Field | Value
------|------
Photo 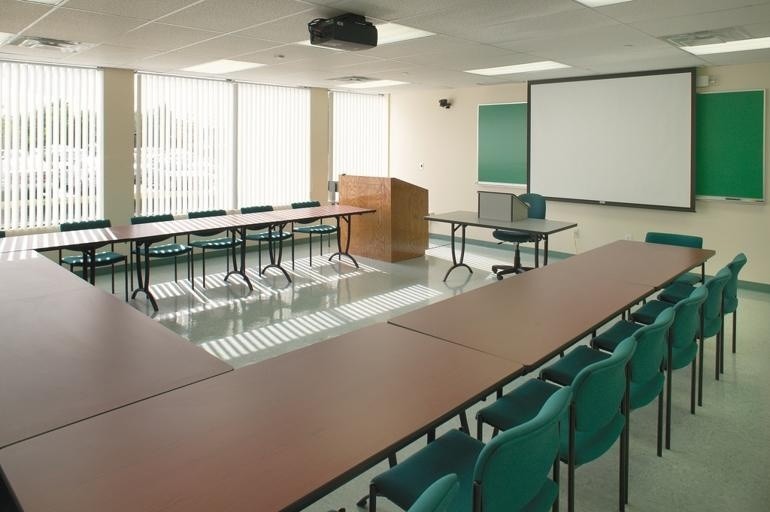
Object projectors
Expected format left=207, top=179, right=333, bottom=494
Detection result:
left=307, top=13, right=378, bottom=52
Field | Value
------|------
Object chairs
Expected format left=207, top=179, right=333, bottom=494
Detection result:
left=292, top=202, right=340, bottom=267
left=59, top=220, right=128, bottom=301
left=188, top=210, right=243, bottom=288
left=130, top=214, right=194, bottom=297
left=241, top=206, right=295, bottom=276
left=492, top=194, right=546, bottom=280
left=646, top=232, right=703, bottom=287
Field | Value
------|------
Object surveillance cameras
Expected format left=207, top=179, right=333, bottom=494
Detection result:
left=439, top=99, right=447, bottom=107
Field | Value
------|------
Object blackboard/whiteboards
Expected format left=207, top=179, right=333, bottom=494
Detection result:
left=477, top=88, right=766, bottom=204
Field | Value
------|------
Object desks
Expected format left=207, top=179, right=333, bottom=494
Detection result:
left=0, top=250, right=233, bottom=449
left=0, top=203, right=377, bottom=311
left=387, top=240, right=717, bottom=401
left=1, top=323, right=525, bottom=512
left=424, top=210, right=577, bottom=282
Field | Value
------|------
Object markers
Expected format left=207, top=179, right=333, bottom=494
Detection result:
left=756, top=201, right=763, bottom=202
left=726, top=198, right=740, bottom=200
